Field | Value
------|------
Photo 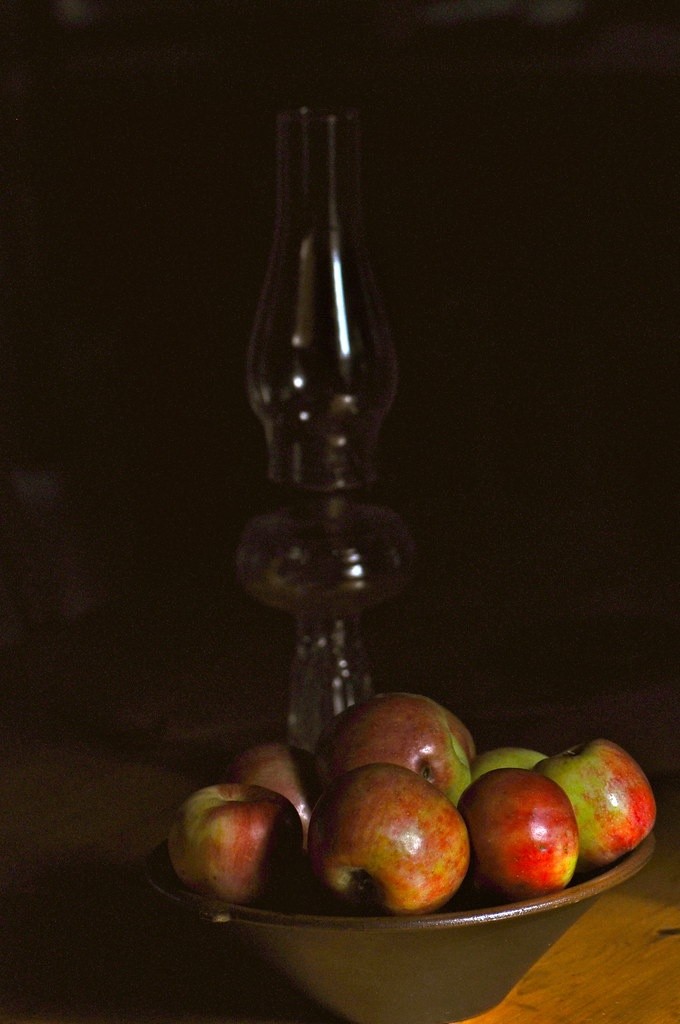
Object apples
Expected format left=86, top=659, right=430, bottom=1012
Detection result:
left=165, top=740, right=317, bottom=904
left=307, top=691, right=656, bottom=919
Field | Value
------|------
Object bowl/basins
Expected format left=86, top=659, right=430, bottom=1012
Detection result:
left=145, top=830, right=657, bottom=1024
